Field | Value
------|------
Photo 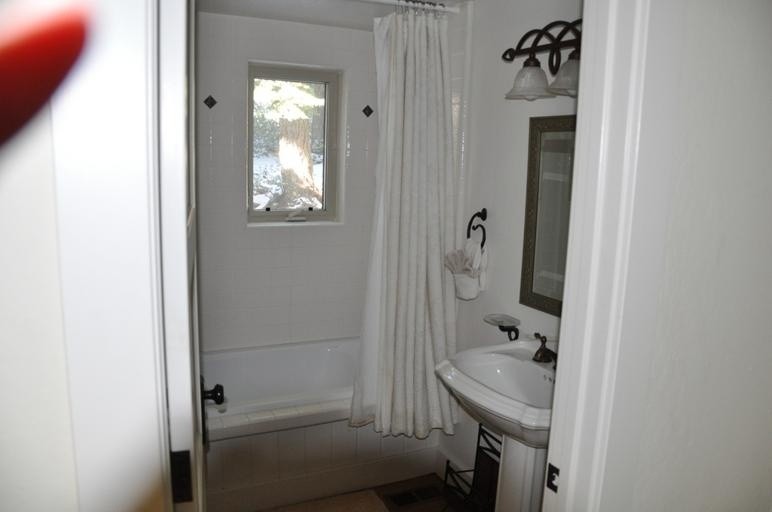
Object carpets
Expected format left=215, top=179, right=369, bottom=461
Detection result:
left=271, top=490, right=389, bottom=512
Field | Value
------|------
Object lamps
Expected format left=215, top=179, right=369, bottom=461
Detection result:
left=501, top=19, right=582, bottom=101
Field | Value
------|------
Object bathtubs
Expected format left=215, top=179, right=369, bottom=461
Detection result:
left=202, top=336, right=442, bottom=508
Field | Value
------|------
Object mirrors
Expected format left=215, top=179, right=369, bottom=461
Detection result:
left=519, top=114, right=577, bottom=318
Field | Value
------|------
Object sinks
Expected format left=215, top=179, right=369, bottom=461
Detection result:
left=434, top=339, right=557, bottom=447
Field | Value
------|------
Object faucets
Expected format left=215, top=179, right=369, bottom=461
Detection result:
left=534, top=332, right=551, bottom=362
